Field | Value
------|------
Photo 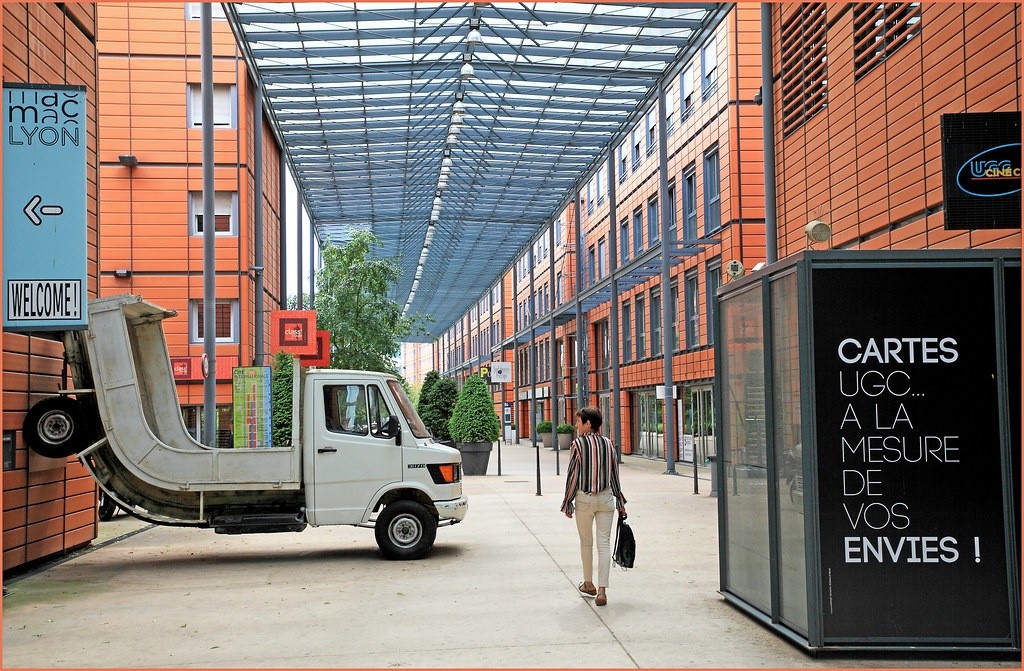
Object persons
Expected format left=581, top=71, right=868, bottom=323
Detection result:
left=559, top=406, right=627, bottom=607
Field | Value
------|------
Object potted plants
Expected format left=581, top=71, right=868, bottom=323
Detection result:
left=537, top=421, right=553, bottom=448
left=417, top=370, right=500, bottom=476
left=556, top=424, right=574, bottom=450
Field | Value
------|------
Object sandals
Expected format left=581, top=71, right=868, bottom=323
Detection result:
left=595, top=593, right=607, bottom=606
left=579, top=581, right=596, bottom=594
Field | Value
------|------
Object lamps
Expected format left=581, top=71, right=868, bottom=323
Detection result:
left=119, top=156, right=138, bottom=166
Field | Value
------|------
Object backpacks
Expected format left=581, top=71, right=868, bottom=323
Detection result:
left=612, top=511, right=635, bottom=571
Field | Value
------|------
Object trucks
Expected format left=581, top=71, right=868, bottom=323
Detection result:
left=22, top=290, right=472, bottom=560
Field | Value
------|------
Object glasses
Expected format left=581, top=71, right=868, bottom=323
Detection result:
left=581, top=408, right=588, bottom=416
